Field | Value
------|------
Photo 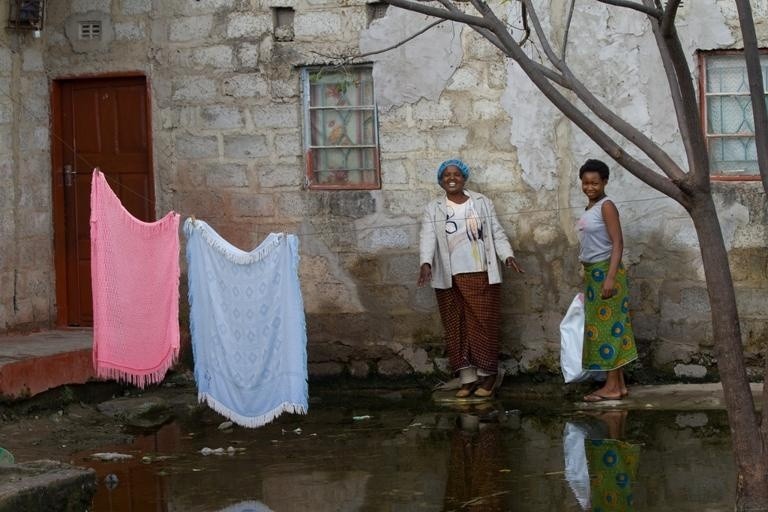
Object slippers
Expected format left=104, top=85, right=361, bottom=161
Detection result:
left=583, top=391, right=629, bottom=402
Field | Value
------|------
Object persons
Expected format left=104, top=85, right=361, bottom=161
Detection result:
left=578, top=408, right=642, bottom=511
left=416, top=159, right=526, bottom=399
left=574, top=157, right=640, bottom=402
left=438, top=426, right=507, bottom=511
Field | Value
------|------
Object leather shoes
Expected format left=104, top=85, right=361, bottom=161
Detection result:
left=474, top=378, right=497, bottom=396
left=455, top=384, right=476, bottom=397
